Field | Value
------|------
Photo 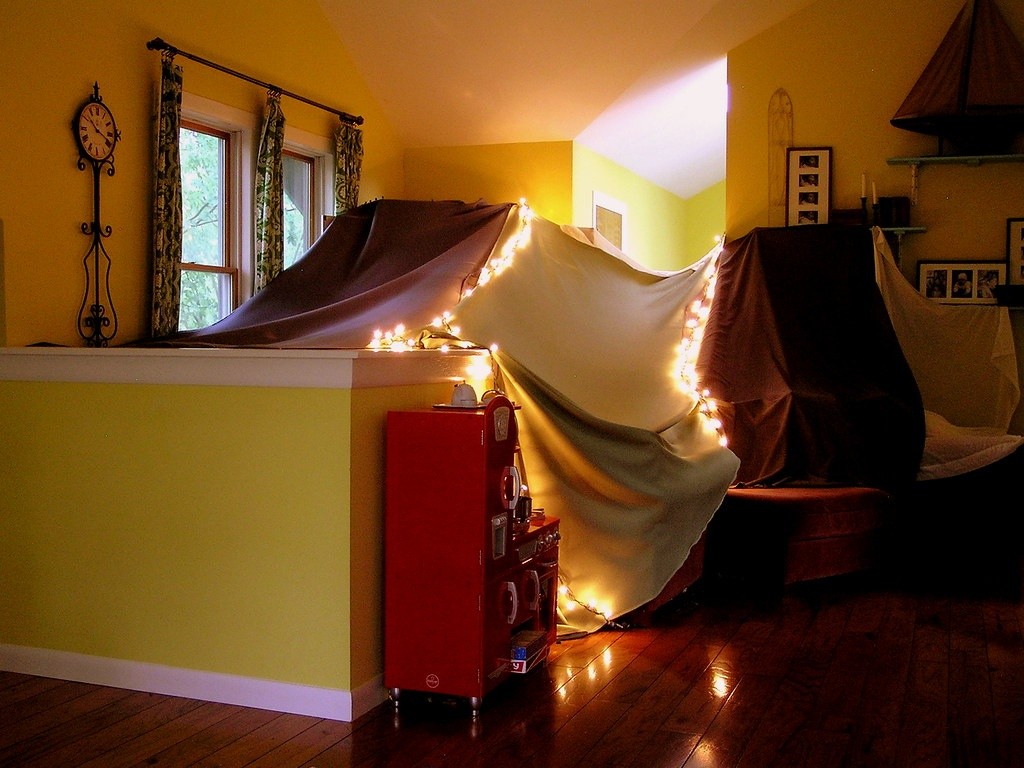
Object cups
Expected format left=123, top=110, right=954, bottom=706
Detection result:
left=516, top=496, right=532, bottom=519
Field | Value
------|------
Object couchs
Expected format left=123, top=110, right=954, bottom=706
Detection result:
left=640, top=484, right=885, bottom=611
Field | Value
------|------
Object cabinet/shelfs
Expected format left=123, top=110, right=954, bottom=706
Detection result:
left=385, top=392, right=562, bottom=728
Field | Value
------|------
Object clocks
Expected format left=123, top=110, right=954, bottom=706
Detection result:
left=71, top=81, right=122, bottom=347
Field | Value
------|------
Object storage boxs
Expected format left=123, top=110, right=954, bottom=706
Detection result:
left=511, top=631, right=548, bottom=674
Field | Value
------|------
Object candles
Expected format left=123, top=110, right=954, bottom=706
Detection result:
left=862, top=172, right=866, bottom=196
left=872, top=180, right=877, bottom=204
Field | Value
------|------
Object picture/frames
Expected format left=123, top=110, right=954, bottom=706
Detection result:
left=784, top=146, right=832, bottom=227
left=916, top=260, right=1009, bottom=306
left=1006, top=217, right=1024, bottom=287
